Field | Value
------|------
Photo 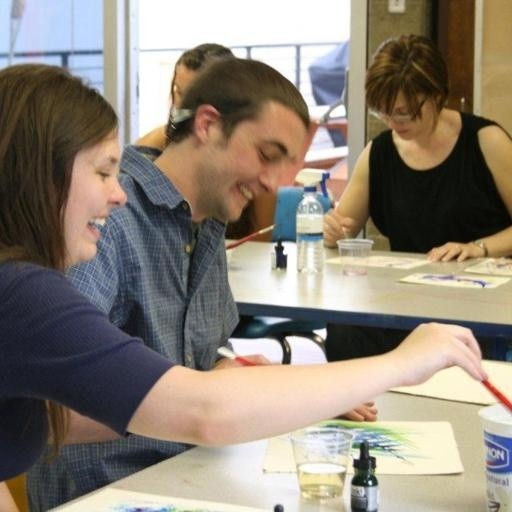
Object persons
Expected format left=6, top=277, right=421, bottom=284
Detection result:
left=134, top=43, right=277, bottom=243
left=0, top=64, right=488, bottom=512
left=323, top=34, right=512, bottom=360
left=26, top=58, right=378, bottom=508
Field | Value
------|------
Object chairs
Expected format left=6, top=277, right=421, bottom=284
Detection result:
left=272, top=188, right=332, bottom=242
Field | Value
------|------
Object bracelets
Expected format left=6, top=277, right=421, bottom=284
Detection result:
left=472, top=240, right=488, bottom=257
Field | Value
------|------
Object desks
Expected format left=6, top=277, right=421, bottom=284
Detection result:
left=47, top=359, right=512, bottom=511
left=222, top=239, right=512, bottom=341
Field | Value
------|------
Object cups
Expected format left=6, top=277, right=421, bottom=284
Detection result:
left=293, top=429, right=354, bottom=500
left=336, top=237, right=371, bottom=277
left=478, top=402, right=511, bottom=512
left=226, top=239, right=238, bottom=263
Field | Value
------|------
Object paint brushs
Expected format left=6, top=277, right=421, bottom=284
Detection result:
left=331, top=202, right=355, bottom=256
left=481, top=379, right=512, bottom=411
left=225, top=223, right=278, bottom=250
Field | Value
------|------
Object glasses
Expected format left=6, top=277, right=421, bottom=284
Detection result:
left=369, top=97, right=429, bottom=122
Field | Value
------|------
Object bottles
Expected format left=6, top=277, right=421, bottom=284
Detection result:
left=294, top=183, right=324, bottom=275
left=352, top=440, right=379, bottom=511
left=270, top=235, right=287, bottom=269
